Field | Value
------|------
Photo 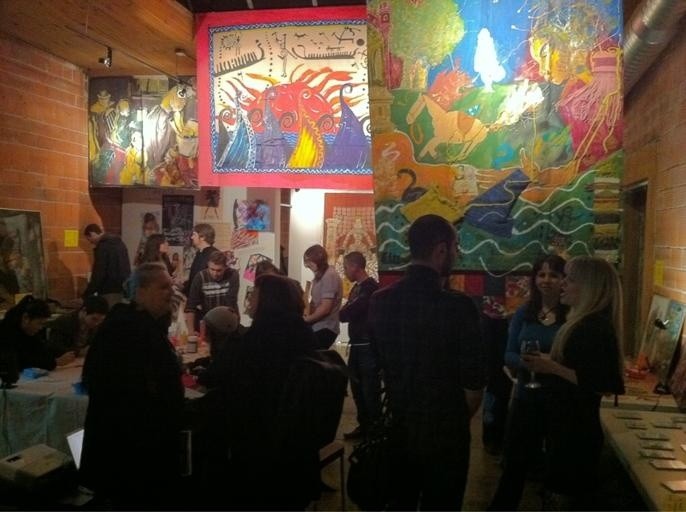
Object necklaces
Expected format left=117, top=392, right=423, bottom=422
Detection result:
left=535, top=304, right=559, bottom=320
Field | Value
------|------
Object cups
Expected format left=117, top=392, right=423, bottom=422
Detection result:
left=520, top=341, right=542, bottom=389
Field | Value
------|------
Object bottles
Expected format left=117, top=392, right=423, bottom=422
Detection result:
left=187, top=336, right=198, bottom=353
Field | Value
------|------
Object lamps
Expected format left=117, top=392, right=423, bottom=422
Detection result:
left=176, top=81, right=186, bottom=99
left=99, top=55, right=111, bottom=69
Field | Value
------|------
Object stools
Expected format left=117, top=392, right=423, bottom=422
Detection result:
left=1, top=444, right=72, bottom=510
left=315, top=439, right=345, bottom=510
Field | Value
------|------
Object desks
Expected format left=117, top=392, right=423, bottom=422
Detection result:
left=4, top=338, right=216, bottom=478
left=599, top=352, right=680, bottom=411
left=597, top=407, right=686, bottom=512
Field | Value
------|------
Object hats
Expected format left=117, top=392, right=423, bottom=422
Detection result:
left=202, top=305, right=238, bottom=335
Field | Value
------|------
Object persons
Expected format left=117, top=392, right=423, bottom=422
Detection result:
left=0, top=295, right=73, bottom=383
left=140, top=231, right=179, bottom=278
left=522, top=258, right=626, bottom=507
left=300, top=242, right=343, bottom=346
left=247, top=260, right=282, bottom=277
left=46, top=294, right=108, bottom=357
left=338, top=247, right=380, bottom=440
left=74, top=224, right=130, bottom=309
left=488, top=254, right=570, bottom=510
left=183, top=250, right=240, bottom=338
left=191, top=302, right=246, bottom=384
left=366, top=211, right=495, bottom=509
left=184, top=223, right=220, bottom=298
left=77, top=260, right=184, bottom=512
left=213, top=272, right=347, bottom=512
